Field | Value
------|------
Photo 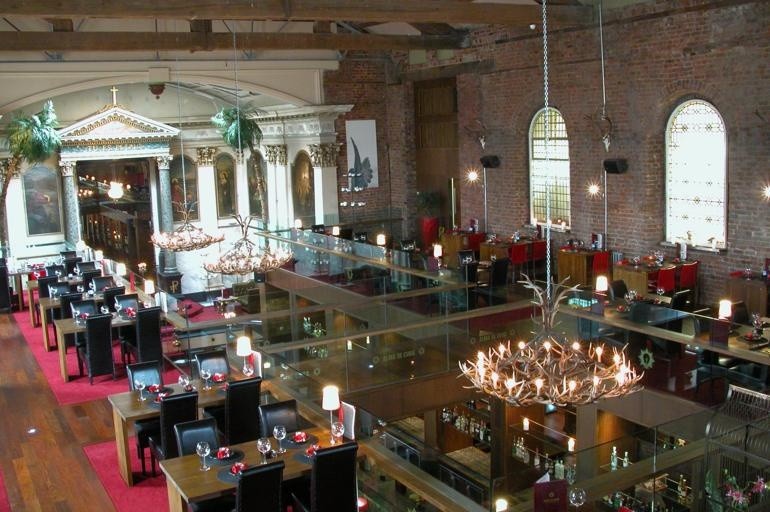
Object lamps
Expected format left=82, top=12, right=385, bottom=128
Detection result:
left=201, top=18, right=294, bottom=275
left=462, top=0, right=645, bottom=412
left=142, top=19, right=230, bottom=250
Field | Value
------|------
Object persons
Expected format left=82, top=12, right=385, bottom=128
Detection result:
left=171, top=178, right=183, bottom=216
left=218, top=171, right=233, bottom=214
left=185, top=191, right=197, bottom=214
left=250, top=171, right=268, bottom=210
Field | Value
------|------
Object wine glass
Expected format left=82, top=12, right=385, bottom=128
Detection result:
left=332, top=421, right=345, bottom=444
left=202, top=369, right=212, bottom=391
left=31, top=255, right=123, bottom=322
left=244, top=364, right=253, bottom=378
left=273, top=425, right=287, bottom=456
left=196, top=442, right=210, bottom=473
left=257, top=436, right=274, bottom=466
left=177, top=376, right=192, bottom=392
left=134, top=379, right=147, bottom=402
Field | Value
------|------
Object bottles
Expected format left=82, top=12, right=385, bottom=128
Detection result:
left=607, top=492, right=672, bottom=511
left=675, top=473, right=688, bottom=501
left=608, top=445, right=618, bottom=466
left=442, top=399, right=491, bottom=442
left=621, top=449, right=629, bottom=467
left=512, top=435, right=577, bottom=485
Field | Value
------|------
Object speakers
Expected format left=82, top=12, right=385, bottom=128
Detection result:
left=480, top=155, right=500, bottom=168
left=604, top=158, right=628, bottom=174
